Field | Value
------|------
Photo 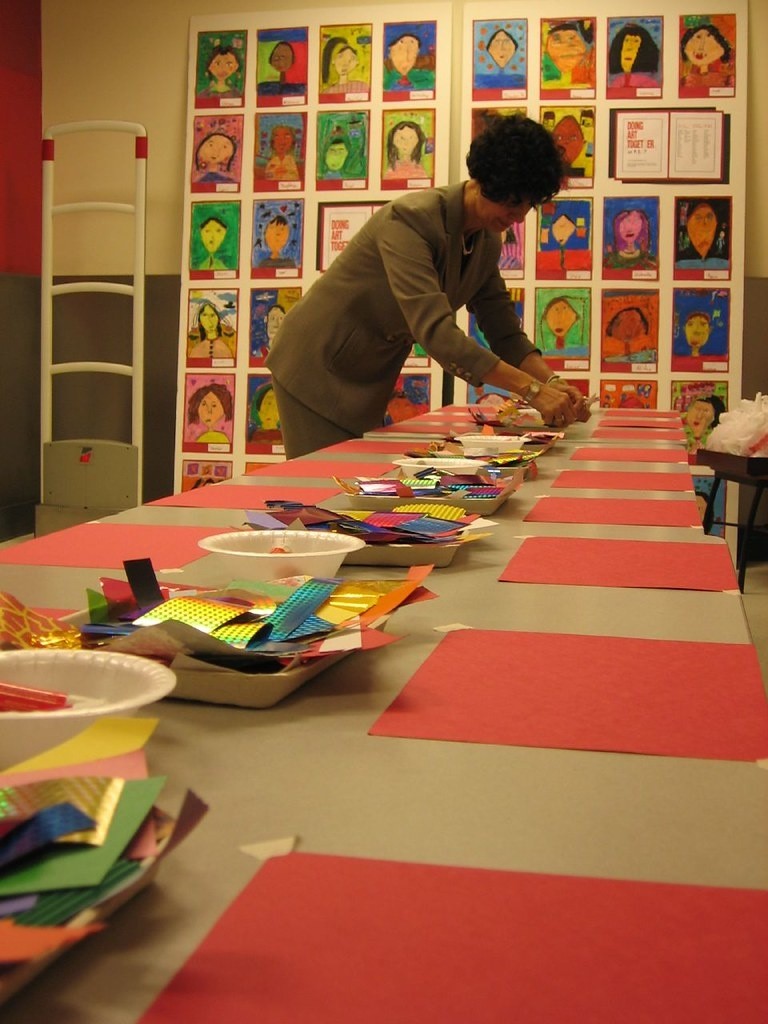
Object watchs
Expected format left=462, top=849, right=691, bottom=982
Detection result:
left=523, top=381, right=541, bottom=405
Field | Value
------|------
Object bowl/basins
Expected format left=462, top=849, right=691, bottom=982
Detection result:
left=198, top=528, right=366, bottom=580
left=392, top=458, right=488, bottom=479
left=0, top=648, right=178, bottom=774
left=454, top=435, right=533, bottom=454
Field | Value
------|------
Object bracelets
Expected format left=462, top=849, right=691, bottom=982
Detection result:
left=547, top=375, right=560, bottom=384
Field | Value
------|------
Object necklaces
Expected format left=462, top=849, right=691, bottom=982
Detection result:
left=461, top=234, right=475, bottom=254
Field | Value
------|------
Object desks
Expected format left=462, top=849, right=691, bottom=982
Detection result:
left=0, top=404, right=768, bottom=1024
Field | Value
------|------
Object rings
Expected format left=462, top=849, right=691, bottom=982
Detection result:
left=583, top=403, right=586, bottom=409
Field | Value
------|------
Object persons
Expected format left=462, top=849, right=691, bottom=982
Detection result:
left=262, top=112, right=592, bottom=466
